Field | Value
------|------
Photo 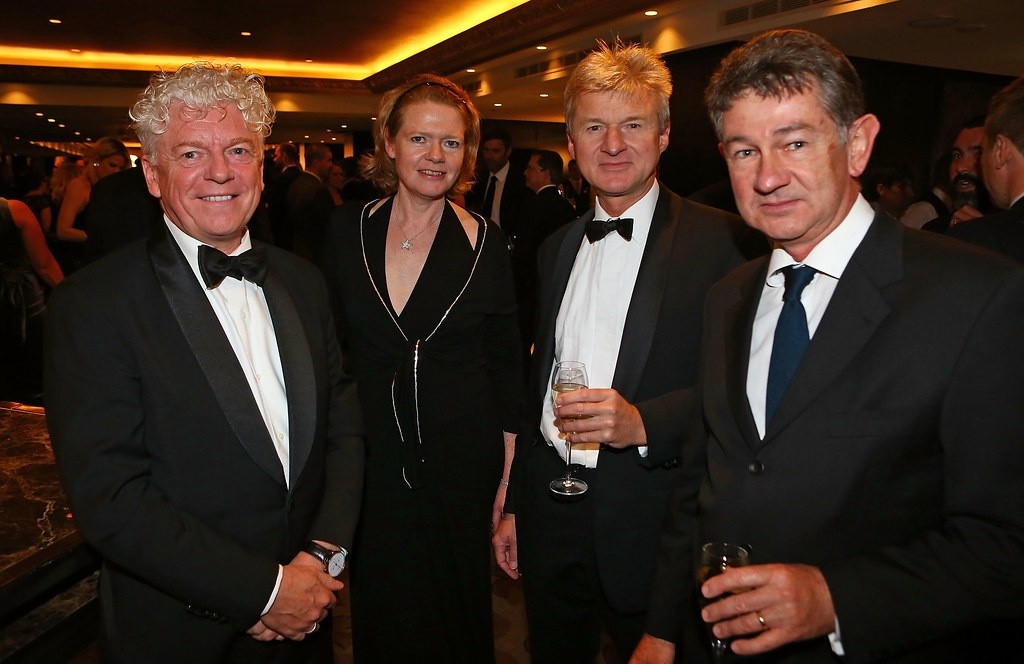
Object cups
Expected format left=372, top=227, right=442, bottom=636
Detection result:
left=509, top=233, right=520, bottom=256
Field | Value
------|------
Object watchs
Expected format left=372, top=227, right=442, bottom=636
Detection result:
left=301, top=540, right=346, bottom=579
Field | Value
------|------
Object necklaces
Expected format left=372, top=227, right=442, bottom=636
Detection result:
left=394, top=195, right=445, bottom=250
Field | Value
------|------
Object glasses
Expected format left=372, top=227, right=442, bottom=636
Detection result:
left=526, top=165, right=543, bottom=171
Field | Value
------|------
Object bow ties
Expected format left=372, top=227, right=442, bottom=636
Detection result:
left=585, top=218, right=633, bottom=244
left=198, top=244, right=269, bottom=290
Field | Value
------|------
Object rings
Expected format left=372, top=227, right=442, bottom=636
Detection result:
left=757, top=610, right=769, bottom=631
left=304, top=623, right=317, bottom=635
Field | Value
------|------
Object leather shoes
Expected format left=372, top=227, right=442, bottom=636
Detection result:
left=33, top=391, right=41, bottom=400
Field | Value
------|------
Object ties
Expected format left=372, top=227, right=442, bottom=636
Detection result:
left=482, top=176, right=498, bottom=218
left=766, top=266, right=818, bottom=434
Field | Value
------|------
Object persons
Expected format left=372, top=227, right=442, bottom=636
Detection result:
left=0, top=135, right=591, bottom=406
left=902, top=114, right=987, bottom=232
left=947, top=72, right=1024, bottom=264
left=868, top=166, right=914, bottom=226
left=494, top=34, right=761, bottom=664
left=42, top=62, right=365, bottom=664
left=627, top=26, right=1024, bottom=664
left=314, top=72, right=520, bottom=663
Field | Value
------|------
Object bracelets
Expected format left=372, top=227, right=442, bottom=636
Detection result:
left=500, top=479, right=508, bottom=485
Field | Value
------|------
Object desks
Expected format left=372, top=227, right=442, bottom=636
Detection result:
left=0, top=398, right=100, bottom=663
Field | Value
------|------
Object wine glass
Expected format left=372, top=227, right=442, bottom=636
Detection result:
left=699, top=542, right=752, bottom=664
left=550, top=361, right=588, bottom=495
